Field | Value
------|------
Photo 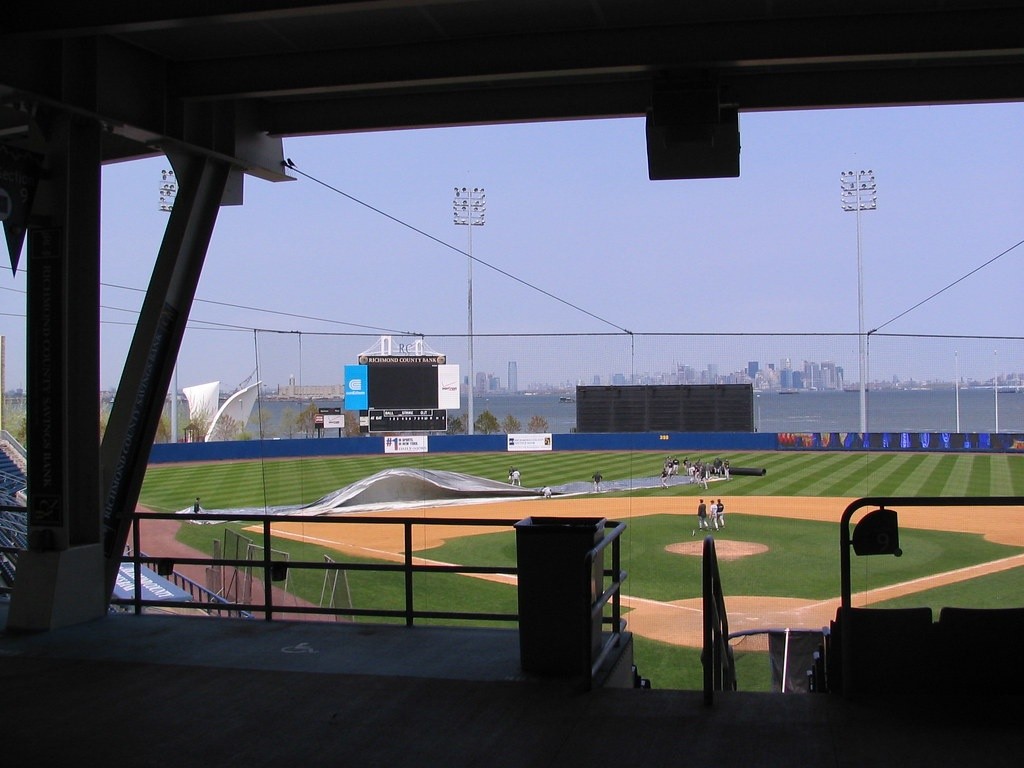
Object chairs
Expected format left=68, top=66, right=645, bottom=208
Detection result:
left=810, top=607, right=1024, bottom=695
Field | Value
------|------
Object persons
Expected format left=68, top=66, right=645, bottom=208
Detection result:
left=194, top=496, right=200, bottom=514
left=592, top=471, right=603, bottom=492
left=660, top=456, right=730, bottom=489
left=698, top=499, right=726, bottom=532
left=508, top=465, right=521, bottom=486
left=541, top=486, right=551, bottom=498
